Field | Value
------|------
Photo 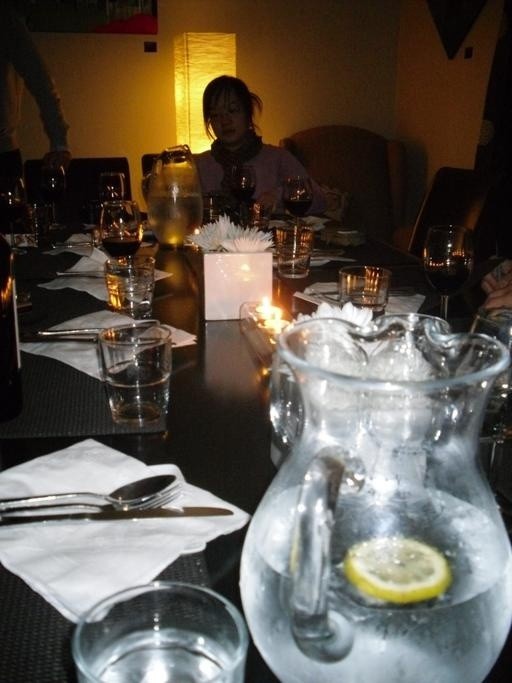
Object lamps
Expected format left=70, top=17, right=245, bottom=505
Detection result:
left=173, top=29, right=238, bottom=153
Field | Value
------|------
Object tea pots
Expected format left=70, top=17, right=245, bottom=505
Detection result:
left=239, top=311, right=512, bottom=683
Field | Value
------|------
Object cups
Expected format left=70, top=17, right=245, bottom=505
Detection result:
left=457, top=309, right=511, bottom=415
left=71, top=580, right=248, bottom=680
left=93, top=323, right=173, bottom=429
left=104, top=255, right=156, bottom=318
left=338, top=265, right=391, bottom=319
left=276, top=224, right=313, bottom=278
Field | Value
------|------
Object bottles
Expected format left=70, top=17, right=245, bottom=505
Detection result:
left=146, top=145, right=204, bottom=249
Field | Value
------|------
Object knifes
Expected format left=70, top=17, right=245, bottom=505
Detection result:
left=1, top=506, right=234, bottom=525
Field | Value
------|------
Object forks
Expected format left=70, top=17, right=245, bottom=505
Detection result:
left=0, top=482, right=183, bottom=511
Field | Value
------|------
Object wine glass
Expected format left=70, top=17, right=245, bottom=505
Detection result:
left=99, top=200, right=143, bottom=265
left=33, top=162, right=68, bottom=232
left=1, top=174, right=27, bottom=256
left=280, top=173, right=314, bottom=225
left=422, top=224, right=473, bottom=323
left=95, top=172, right=125, bottom=200
left=228, top=163, right=256, bottom=220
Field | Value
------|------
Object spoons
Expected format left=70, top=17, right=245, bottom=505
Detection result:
left=1, top=473, right=176, bottom=507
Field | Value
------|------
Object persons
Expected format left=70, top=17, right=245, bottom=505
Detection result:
left=195, top=75, right=328, bottom=216
left=0, top=0, right=72, bottom=291
left=480, top=259, right=512, bottom=310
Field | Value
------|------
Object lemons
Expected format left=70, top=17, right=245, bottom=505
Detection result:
left=343, top=535, right=452, bottom=606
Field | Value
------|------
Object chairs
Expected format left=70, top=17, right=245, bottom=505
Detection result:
left=276, top=124, right=407, bottom=270
left=141, top=152, right=223, bottom=233
left=22, top=156, right=131, bottom=232
left=408, top=164, right=502, bottom=272
